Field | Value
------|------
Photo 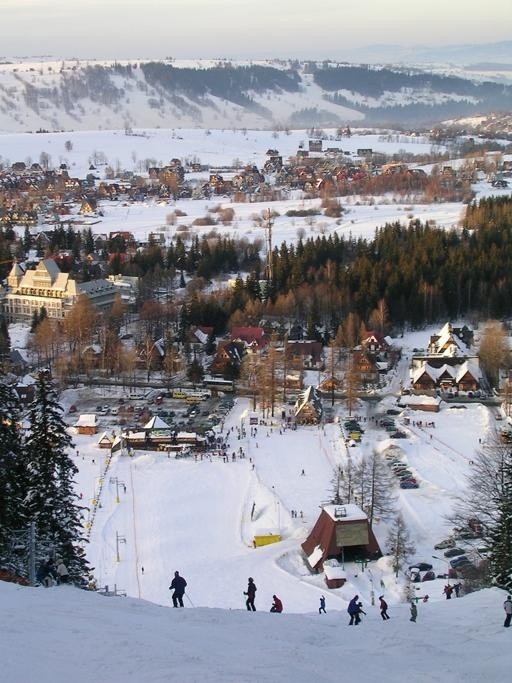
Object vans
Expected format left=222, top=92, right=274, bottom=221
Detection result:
left=127, top=394, right=142, bottom=399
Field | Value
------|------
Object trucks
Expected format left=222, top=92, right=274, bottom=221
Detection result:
left=453, top=519, right=484, bottom=538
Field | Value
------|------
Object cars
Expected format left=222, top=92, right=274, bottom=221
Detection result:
left=420, top=571, right=435, bottom=581
left=158, top=410, right=176, bottom=423
left=96, top=404, right=118, bottom=415
left=409, top=562, right=432, bottom=571
left=344, top=420, right=364, bottom=439
left=182, top=406, right=209, bottom=424
left=434, top=539, right=472, bottom=571
left=379, top=418, right=406, bottom=439
left=391, top=463, right=418, bottom=488
left=70, top=406, right=75, bottom=412
left=386, top=409, right=399, bottom=415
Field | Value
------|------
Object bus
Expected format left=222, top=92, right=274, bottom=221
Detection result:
left=172, top=392, right=207, bottom=404
left=204, top=380, right=233, bottom=392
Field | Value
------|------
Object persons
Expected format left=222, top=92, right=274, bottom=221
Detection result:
left=269, top=594, right=283, bottom=613
left=243, top=576, right=258, bottom=611
left=454, top=583, right=459, bottom=597
left=194, top=423, right=286, bottom=469
left=444, top=585, right=451, bottom=599
left=378, top=593, right=390, bottom=621
left=357, top=601, right=366, bottom=621
left=503, top=594, right=511, bottom=627
left=347, top=594, right=360, bottom=625
left=409, top=601, right=416, bottom=622
left=318, top=594, right=326, bottom=613
left=169, top=570, right=187, bottom=607
